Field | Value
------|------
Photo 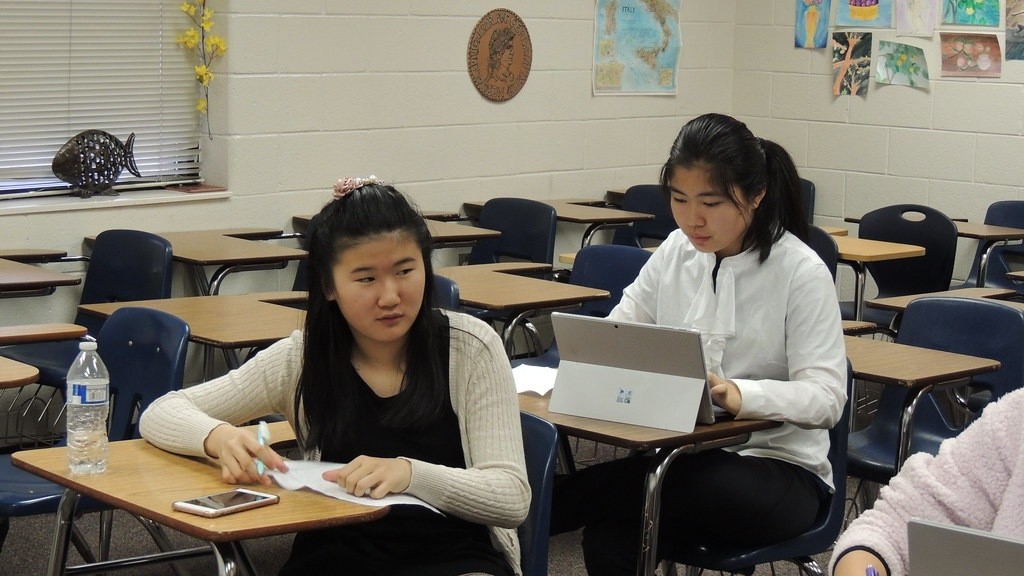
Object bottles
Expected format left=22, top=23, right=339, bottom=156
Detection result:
left=67, top=342, right=110, bottom=474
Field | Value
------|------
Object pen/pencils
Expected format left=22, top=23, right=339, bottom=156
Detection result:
left=254, top=421, right=271, bottom=476
left=866, top=565, right=876, bottom=576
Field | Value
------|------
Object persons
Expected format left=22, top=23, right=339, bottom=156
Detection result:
left=136, top=177, right=533, bottom=576
left=827, top=382, right=1024, bottom=576
left=550, top=113, right=851, bottom=576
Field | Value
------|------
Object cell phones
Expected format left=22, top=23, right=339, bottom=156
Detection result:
left=171, top=488, right=279, bottom=517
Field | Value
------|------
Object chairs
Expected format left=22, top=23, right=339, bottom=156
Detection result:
left=848, top=296, right=1024, bottom=496
left=0, top=229, right=174, bottom=449
left=0, top=307, right=259, bottom=576
left=838, top=204, right=958, bottom=336
left=660, top=356, right=852, bottom=576
left=580, top=183, right=681, bottom=250
left=511, top=245, right=652, bottom=371
left=782, top=176, right=837, bottom=284
left=468, top=197, right=557, bottom=357
left=949, top=199, right=1024, bottom=301
left=520, top=410, right=558, bottom=576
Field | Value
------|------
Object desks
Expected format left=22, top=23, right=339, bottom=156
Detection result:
left=0, top=201, right=1024, bottom=576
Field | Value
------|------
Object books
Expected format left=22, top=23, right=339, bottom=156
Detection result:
left=163, top=184, right=228, bottom=193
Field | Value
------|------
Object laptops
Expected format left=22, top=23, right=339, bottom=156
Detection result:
left=551, top=312, right=735, bottom=425
left=907, top=518, right=1024, bottom=576
